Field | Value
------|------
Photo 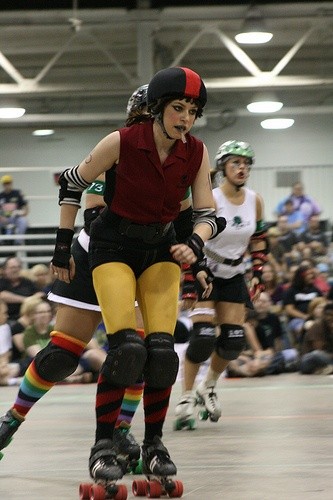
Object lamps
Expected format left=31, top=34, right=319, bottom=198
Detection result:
left=246, top=91, right=284, bottom=113
left=235, top=5, right=274, bottom=43
left=0, top=98, right=26, bottom=118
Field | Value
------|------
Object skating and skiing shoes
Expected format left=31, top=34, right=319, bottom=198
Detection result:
left=78, top=445, right=128, bottom=500
left=112, top=428, right=144, bottom=475
left=132, top=440, right=184, bottom=498
left=0, top=410, right=21, bottom=462
left=196, top=382, right=222, bottom=422
left=173, top=394, right=195, bottom=429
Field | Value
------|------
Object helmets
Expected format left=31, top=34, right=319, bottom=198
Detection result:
left=127, top=84, right=149, bottom=116
left=215, top=140, right=255, bottom=166
left=147, top=67, right=208, bottom=107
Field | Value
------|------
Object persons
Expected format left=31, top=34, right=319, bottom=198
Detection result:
left=0, top=83, right=215, bottom=475
left=0, top=175, right=28, bottom=245
left=50, top=67, right=218, bottom=500
left=174, top=140, right=265, bottom=431
left=173, top=182, right=333, bottom=377
left=0, top=256, right=110, bottom=385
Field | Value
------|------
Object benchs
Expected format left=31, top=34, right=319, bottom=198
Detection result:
left=0, top=234, right=79, bottom=270
